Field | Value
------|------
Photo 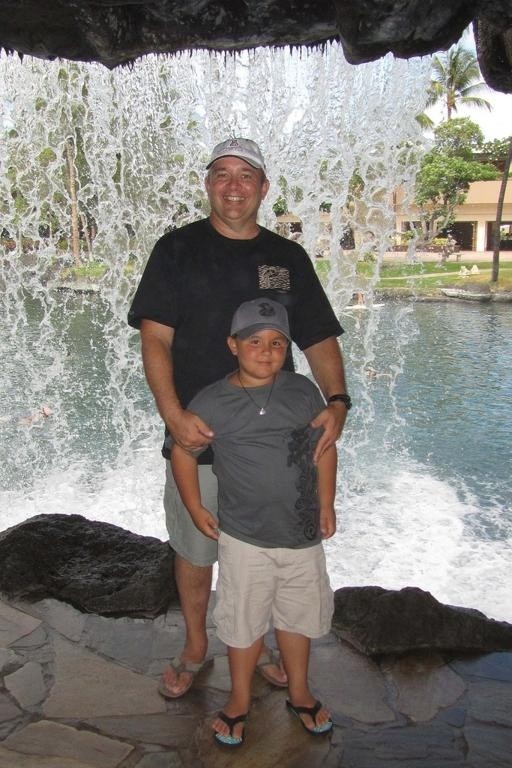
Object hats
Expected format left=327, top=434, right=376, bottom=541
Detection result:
left=230, top=297, right=291, bottom=342
left=205, top=138, right=264, bottom=170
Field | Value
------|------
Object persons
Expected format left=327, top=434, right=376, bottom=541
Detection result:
left=125, top=135, right=354, bottom=701
left=168, top=295, right=338, bottom=746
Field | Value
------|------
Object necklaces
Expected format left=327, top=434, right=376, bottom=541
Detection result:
left=234, top=369, right=277, bottom=416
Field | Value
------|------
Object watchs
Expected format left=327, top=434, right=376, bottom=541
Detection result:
left=326, top=392, right=353, bottom=410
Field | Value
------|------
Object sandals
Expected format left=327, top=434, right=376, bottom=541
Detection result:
left=285, top=696, right=333, bottom=734
left=159, top=655, right=215, bottom=698
left=213, top=711, right=248, bottom=747
left=255, top=651, right=288, bottom=687
